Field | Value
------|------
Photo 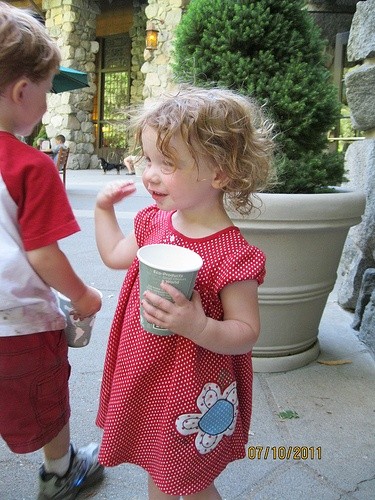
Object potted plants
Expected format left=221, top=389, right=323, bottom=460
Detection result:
left=166, top=0, right=369, bottom=374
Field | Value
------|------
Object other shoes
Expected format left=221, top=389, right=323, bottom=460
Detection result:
left=126, top=172, right=135, bottom=175
left=38, top=441, right=105, bottom=500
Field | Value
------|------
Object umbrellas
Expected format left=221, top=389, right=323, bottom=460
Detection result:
left=47, top=65, right=90, bottom=94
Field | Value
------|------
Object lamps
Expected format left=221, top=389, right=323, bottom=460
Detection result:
left=145, top=15, right=167, bottom=52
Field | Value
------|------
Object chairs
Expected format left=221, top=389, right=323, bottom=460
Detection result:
left=54, top=146, right=70, bottom=191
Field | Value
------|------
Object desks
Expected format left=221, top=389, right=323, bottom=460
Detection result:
left=39, top=148, right=54, bottom=154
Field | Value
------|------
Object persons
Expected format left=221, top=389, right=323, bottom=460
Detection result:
left=95, top=85, right=286, bottom=500
left=0, top=1, right=105, bottom=500
left=124, top=155, right=136, bottom=175
left=42, top=134, right=67, bottom=170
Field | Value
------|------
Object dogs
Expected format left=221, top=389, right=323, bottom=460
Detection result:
left=96, top=156, right=127, bottom=176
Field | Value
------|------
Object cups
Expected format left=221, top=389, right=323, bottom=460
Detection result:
left=137, top=244, right=204, bottom=336
left=58, top=285, right=102, bottom=348
